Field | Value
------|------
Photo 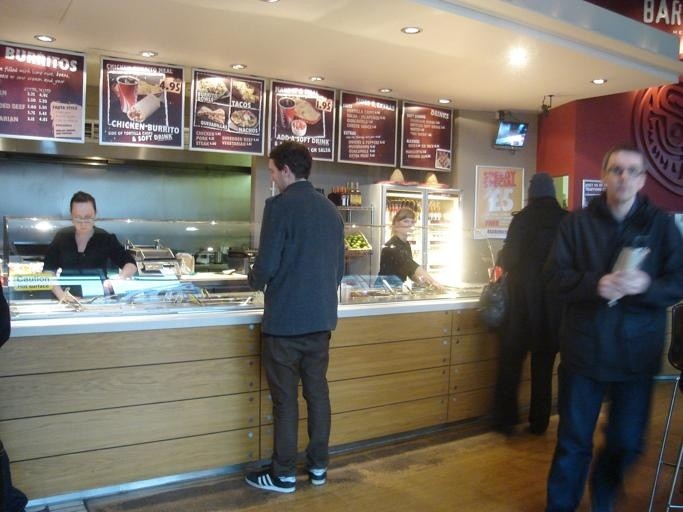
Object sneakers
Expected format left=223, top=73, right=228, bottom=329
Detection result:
left=244, top=472, right=297, bottom=493
left=481, top=283, right=506, bottom=326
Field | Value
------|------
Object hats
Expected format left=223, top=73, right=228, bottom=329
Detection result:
left=529, top=173, right=555, bottom=197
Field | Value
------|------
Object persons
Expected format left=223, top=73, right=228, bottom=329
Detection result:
left=0, top=281, right=29, bottom=512
left=541, top=144, right=682, bottom=511
left=42, top=191, right=139, bottom=304
left=374, top=208, right=438, bottom=289
left=476, top=172, right=574, bottom=435
left=242, top=140, right=345, bottom=494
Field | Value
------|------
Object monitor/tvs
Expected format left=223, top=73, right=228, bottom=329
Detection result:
left=492, top=120, right=528, bottom=149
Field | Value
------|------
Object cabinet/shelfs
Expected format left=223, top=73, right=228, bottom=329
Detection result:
left=335, top=204, right=374, bottom=289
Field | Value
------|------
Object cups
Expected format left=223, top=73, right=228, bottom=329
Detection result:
left=117, top=75, right=140, bottom=113
left=278, top=96, right=298, bottom=128
left=212, top=250, right=224, bottom=265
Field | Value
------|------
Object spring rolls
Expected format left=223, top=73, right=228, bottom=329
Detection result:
left=127, top=93, right=161, bottom=123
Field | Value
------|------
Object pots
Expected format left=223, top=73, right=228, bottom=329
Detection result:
left=227, top=243, right=259, bottom=275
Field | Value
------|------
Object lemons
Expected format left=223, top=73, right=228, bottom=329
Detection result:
left=345, top=232, right=369, bottom=250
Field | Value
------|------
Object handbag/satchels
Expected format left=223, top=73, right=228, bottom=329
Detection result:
left=309, top=468, right=326, bottom=486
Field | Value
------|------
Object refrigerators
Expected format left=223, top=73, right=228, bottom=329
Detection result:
left=348, top=182, right=465, bottom=290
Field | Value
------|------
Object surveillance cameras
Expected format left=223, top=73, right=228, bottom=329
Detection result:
left=542, top=104, right=551, bottom=114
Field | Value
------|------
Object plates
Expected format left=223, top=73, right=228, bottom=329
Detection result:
left=197, top=77, right=228, bottom=100
left=229, top=110, right=259, bottom=131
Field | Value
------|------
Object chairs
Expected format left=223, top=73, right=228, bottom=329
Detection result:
left=649, top=303, right=683, bottom=510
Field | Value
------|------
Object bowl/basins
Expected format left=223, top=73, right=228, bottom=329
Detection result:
left=290, top=118, right=306, bottom=137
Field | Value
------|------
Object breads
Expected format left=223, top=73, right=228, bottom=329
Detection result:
left=288, top=96, right=322, bottom=125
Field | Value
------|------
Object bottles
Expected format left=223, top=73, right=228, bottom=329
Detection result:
left=329, top=179, right=362, bottom=207
left=403, top=226, right=443, bottom=254
left=386, top=197, right=441, bottom=223
